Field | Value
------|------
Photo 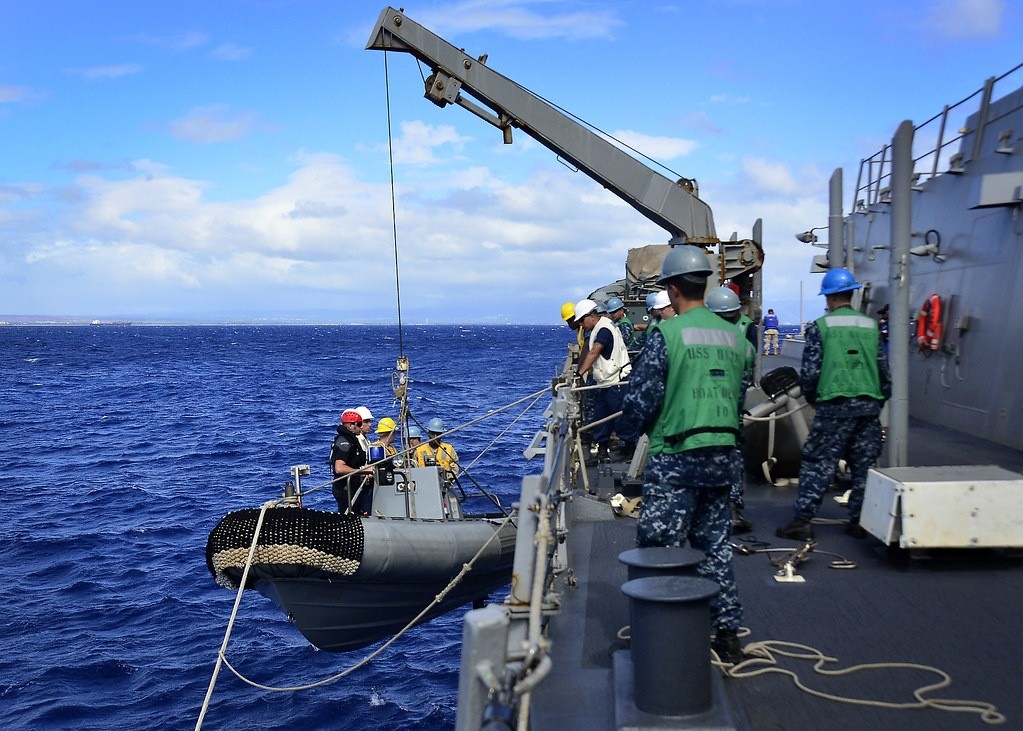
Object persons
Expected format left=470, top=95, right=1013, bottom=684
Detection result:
left=775, top=267, right=891, bottom=541
left=328, top=412, right=375, bottom=512
left=411, top=418, right=459, bottom=485
left=616, top=246, right=756, bottom=664
left=705, top=287, right=759, bottom=528
left=574, top=299, right=632, bottom=464
left=402, top=426, right=424, bottom=458
left=606, top=298, right=634, bottom=347
left=561, top=302, right=591, bottom=348
left=369, top=418, right=399, bottom=457
left=631, top=293, right=661, bottom=352
left=337, top=407, right=374, bottom=515
left=762, top=309, right=779, bottom=356
left=653, top=290, right=679, bottom=322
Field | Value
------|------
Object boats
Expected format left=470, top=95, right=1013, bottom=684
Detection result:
left=206, top=462, right=522, bottom=654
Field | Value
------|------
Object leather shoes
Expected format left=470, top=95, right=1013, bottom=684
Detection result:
left=776, top=517, right=814, bottom=542
left=730, top=506, right=750, bottom=531
left=843, top=517, right=868, bottom=539
left=584, top=444, right=611, bottom=468
left=624, top=442, right=637, bottom=460
left=709, top=628, right=741, bottom=666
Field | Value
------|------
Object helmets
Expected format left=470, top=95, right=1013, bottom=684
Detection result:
left=561, top=301, right=576, bottom=321
left=706, top=286, right=742, bottom=313
left=817, top=267, right=861, bottom=296
left=374, top=418, right=400, bottom=434
left=355, top=406, right=374, bottom=421
left=646, top=291, right=658, bottom=312
left=573, top=298, right=598, bottom=322
left=402, top=426, right=423, bottom=438
left=606, top=297, right=625, bottom=312
left=426, top=418, right=447, bottom=433
left=340, top=408, right=363, bottom=422
left=594, top=303, right=607, bottom=314
left=652, top=290, right=672, bottom=310
left=656, top=245, right=714, bottom=287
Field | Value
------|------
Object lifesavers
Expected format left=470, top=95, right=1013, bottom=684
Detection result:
left=916, top=292, right=941, bottom=350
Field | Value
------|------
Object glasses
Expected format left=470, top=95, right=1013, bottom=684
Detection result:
left=350, top=422, right=362, bottom=427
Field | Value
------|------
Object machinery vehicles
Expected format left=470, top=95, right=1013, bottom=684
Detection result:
left=366, top=6, right=768, bottom=399
